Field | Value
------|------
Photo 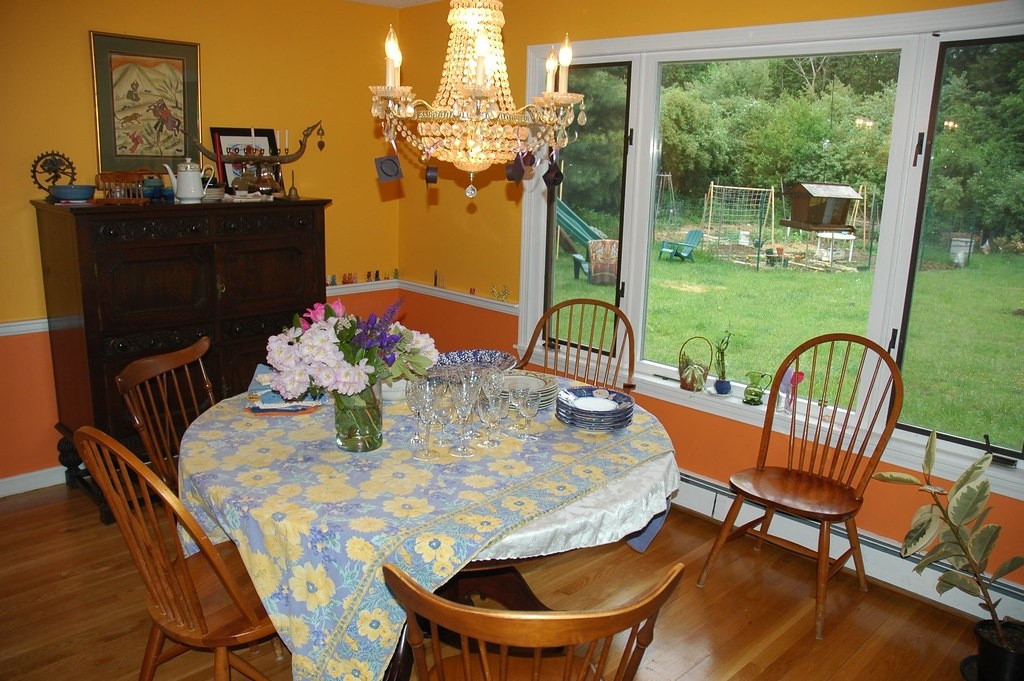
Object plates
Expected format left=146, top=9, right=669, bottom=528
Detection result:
left=554, top=386, right=635, bottom=430
left=426, top=350, right=517, bottom=381
left=478, top=369, right=560, bottom=411
left=381, top=380, right=407, bottom=401
left=203, top=188, right=225, bottom=203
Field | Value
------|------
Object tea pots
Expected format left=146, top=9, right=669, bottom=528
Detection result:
left=162, top=157, right=214, bottom=204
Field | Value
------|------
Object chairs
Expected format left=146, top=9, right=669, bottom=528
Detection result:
left=572, top=238, right=620, bottom=285
left=72, top=425, right=284, bottom=681
left=514, top=298, right=635, bottom=395
left=115, top=336, right=216, bottom=519
left=382, top=555, right=686, bottom=681
left=658, top=229, right=703, bottom=263
left=697, top=331, right=904, bottom=640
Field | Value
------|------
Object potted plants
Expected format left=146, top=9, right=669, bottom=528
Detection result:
left=712, top=333, right=735, bottom=394
left=870, top=429, right=1024, bottom=681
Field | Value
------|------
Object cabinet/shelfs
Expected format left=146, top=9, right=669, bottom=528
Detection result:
left=29, top=196, right=332, bottom=524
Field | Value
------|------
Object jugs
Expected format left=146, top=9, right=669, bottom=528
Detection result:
left=753, top=238, right=766, bottom=248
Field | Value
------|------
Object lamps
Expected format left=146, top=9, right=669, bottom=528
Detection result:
left=368, top=0, right=587, bottom=199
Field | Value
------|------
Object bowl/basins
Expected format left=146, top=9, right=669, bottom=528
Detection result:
left=127, top=188, right=152, bottom=198
left=49, top=185, right=97, bottom=202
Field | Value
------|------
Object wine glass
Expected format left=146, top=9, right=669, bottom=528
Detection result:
left=449, top=363, right=512, bottom=458
left=406, top=366, right=455, bottom=461
left=509, top=382, right=541, bottom=442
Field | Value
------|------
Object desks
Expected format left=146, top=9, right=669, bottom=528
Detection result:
left=176, top=374, right=682, bottom=681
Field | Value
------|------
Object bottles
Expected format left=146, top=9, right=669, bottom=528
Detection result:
left=777, top=368, right=794, bottom=414
left=116, top=181, right=120, bottom=197
left=743, top=372, right=772, bottom=406
left=143, top=176, right=163, bottom=199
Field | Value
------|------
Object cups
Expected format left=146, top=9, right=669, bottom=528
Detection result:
left=161, top=186, right=174, bottom=201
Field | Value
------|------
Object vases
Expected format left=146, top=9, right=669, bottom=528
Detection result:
left=330, top=377, right=384, bottom=452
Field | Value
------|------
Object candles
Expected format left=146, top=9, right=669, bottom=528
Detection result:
left=251, top=126, right=255, bottom=148
left=286, top=129, right=289, bottom=149
left=277, top=129, right=280, bottom=149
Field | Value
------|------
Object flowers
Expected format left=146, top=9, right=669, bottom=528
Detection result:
left=266, top=299, right=439, bottom=446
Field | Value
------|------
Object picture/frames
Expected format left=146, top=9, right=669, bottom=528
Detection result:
left=208, top=126, right=285, bottom=194
left=89, top=29, right=204, bottom=174
